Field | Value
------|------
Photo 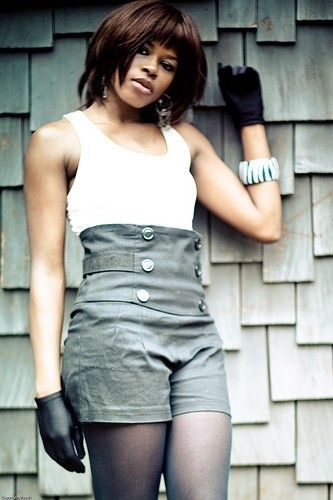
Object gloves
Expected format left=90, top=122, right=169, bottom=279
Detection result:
left=34, top=390, right=86, bottom=473
left=217, top=62, right=266, bottom=128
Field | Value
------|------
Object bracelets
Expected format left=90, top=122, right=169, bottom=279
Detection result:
left=239, top=156, right=281, bottom=184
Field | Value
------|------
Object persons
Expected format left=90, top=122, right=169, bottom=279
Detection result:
left=23, top=0, right=283, bottom=500
